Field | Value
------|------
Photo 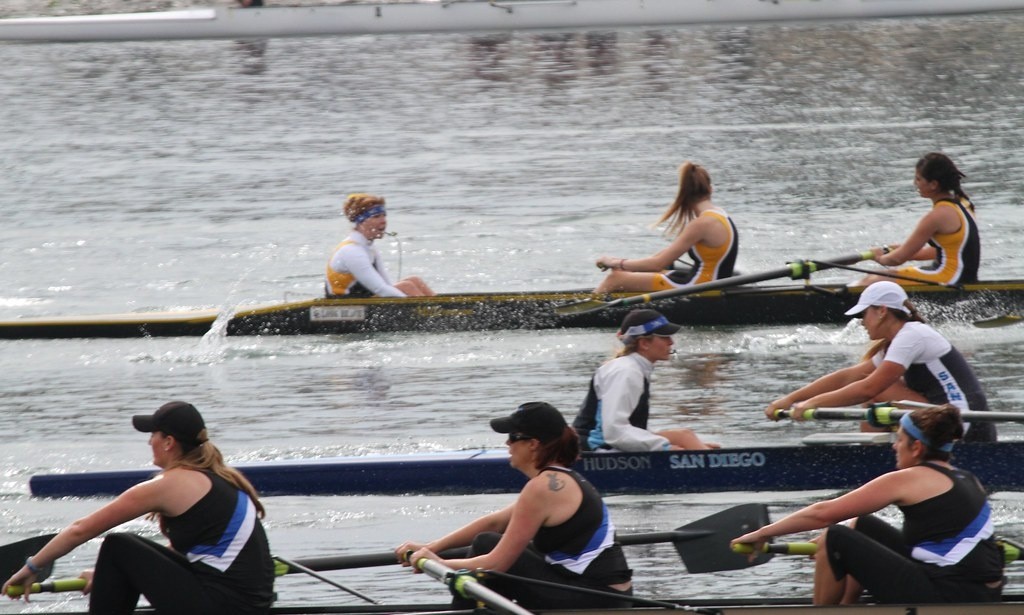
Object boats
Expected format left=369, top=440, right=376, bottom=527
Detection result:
left=0, top=594, right=1024, bottom=615
left=0, top=271, right=1024, bottom=341
left=31, top=434, right=1023, bottom=500
left=1, top=0, right=1024, bottom=43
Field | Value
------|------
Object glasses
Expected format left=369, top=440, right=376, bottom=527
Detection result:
left=508, top=434, right=537, bottom=442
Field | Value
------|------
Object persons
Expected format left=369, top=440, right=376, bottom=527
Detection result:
left=0, top=400, right=278, bottom=615
left=858, top=152, right=981, bottom=284
left=325, top=193, right=435, bottom=297
left=392, top=401, right=634, bottom=606
left=594, top=161, right=738, bottom=293
left=570, top=309, right=722, bottom=454
left=764, top=280, right=998, bottom=445
left=730, top=403, right=1008, bottom=605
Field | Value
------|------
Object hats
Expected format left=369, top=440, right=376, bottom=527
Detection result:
left=132, top=402, right=208, bottom=447
left=490, top=401, right=566, bottom=445
left=844, top=280, right=912, bottom=315
left=619, top=311, right=682, bottom=337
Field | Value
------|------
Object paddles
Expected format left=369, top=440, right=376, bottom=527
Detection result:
left=0, top=533, right=59, bottom=596
left=7, top=501, right=772, bottom=597
left=732, top=537, right=1024, bottom=563
left=404, top=550, right=535, bottom=615
left=554, top=246, right=893, bottom=316
left=773, top=407, right=1024, bottom=422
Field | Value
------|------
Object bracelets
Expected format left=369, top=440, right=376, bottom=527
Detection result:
left=26, top=555, right=44, bottom=573
left=620, top=259, right=625, bottom=270
